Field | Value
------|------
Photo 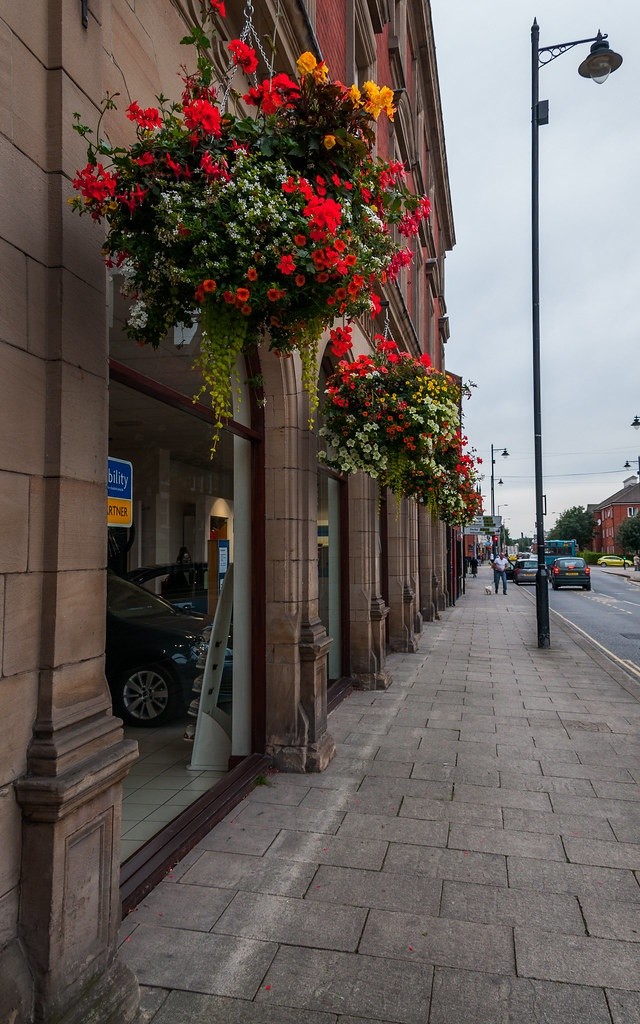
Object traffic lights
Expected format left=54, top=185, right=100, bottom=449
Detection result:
left=493, top=537, right=498, bottom=546
left=622, top=556, right=626, bottom=560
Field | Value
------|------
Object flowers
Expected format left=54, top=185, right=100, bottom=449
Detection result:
left=70, top=0, right=487, bottom=532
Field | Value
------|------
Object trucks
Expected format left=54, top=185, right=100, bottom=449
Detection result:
left=508, top=546, right=519, bottom=559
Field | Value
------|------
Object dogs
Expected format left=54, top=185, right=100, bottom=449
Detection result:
left=485, top=586, right=492, bottom=595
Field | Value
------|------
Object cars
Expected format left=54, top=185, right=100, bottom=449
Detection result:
left=130, top=561, right=207, bottom=614
left=104, top=569, right=233, bottom=724
left=508, top=554, right=517, bottom=561
left=549, top=557, right=591, bottom=591
left=522, top=554, right=530, bottom=559
left=506, top=559, right=537, bottom=585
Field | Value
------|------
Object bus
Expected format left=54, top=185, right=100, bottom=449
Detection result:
left=542, top=539, right=576, bottom=566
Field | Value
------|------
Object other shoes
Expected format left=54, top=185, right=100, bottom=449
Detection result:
left=503, top=592, right=508, bottom=595
left=495, top=589, right=498, bottom=594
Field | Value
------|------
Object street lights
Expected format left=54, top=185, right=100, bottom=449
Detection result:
left=497, top=504, right=508, bottom=516
left=622, top=457, right=640, bottom=482
left=480, top=495, right=487, bottom=515
left=491, top=478, right=505, bottom=516
left=490, top=445, right=509, bottom=517
left=528, top=19, right=622, bottom=649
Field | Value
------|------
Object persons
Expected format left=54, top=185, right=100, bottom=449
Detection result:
left=470, top=557, right=478, bottom=577
left=492, top=553, right=508, bottom=595
left=634, top=555, right=640, bottom=571
left=484, top=535, right=492, bottom=546
left=490, top=552, right=494, bottom=565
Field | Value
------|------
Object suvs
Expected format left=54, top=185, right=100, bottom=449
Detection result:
left=597, top=555, right=633, bottom=567
